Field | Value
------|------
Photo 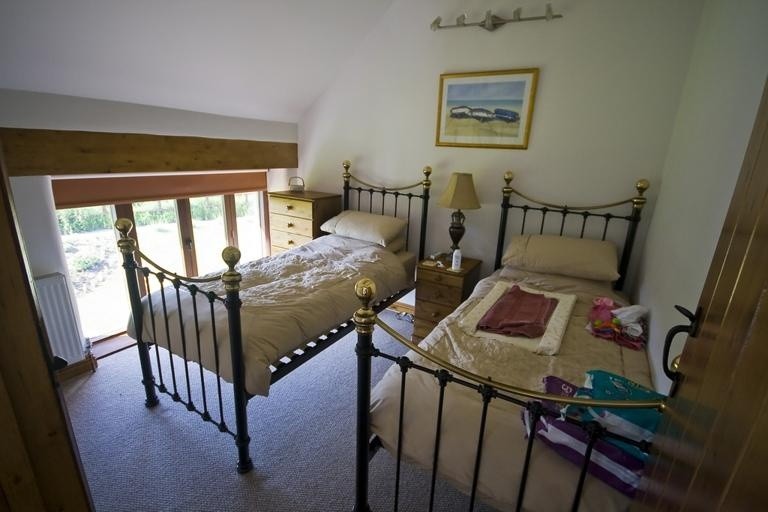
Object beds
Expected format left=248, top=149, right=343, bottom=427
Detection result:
left=345, top=173, right=662, bottom=508
left=117, top=162, right=431, bottom=476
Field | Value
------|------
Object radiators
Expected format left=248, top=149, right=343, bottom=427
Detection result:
left=33, top=271, right=86, bottom=368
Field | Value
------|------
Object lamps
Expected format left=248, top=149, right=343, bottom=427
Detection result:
left=437, top=171, right=482, bottom=262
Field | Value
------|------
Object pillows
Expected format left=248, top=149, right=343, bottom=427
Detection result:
left=501, top=233, right=621, bottom=299
left=320, top=207, right=409, bottom=254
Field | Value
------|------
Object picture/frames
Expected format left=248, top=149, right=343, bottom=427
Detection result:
left=435, top=69, right=539, bottom=150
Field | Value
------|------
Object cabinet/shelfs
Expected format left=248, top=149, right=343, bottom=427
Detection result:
left=266, top=190, right=342, bottom=260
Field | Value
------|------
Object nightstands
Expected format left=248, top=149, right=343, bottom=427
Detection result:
left=410, top=254, right=482, bottom=344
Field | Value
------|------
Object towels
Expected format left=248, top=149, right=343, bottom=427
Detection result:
left=456, top=283, right=576, bottom=357
